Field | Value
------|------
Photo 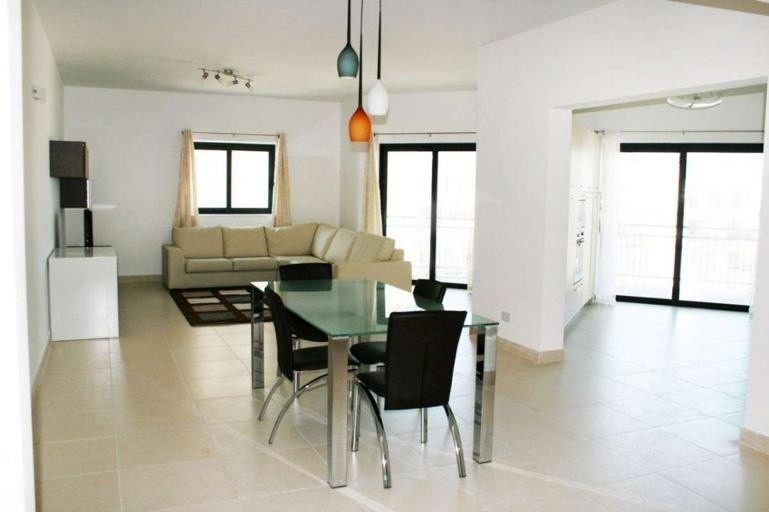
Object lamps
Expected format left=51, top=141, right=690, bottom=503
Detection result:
left=336, top=0, right=391, bottom=142
left=198, top=68, right=255, bottom=92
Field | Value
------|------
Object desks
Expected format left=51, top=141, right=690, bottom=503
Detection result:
left=249, top=278, right=500, bottom=489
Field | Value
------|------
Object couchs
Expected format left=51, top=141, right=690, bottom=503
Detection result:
left=162, top=222, right=412, bottom=292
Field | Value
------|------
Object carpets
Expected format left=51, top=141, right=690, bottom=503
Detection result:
left=170, top=286, right=272, bottom=327
